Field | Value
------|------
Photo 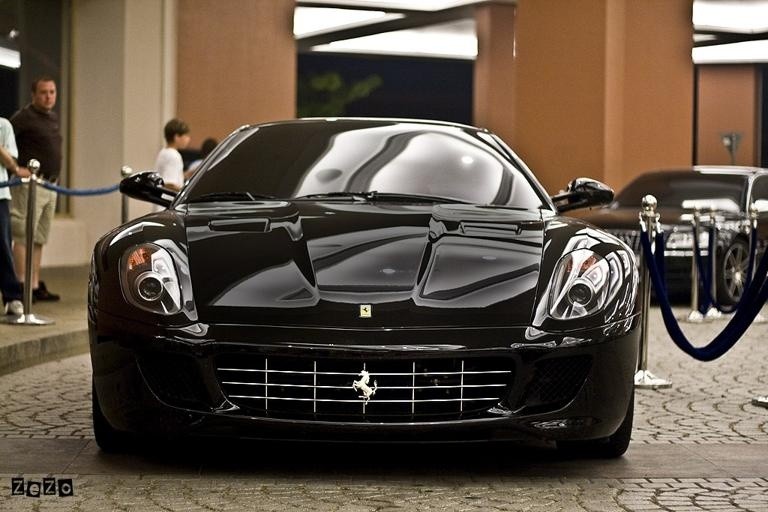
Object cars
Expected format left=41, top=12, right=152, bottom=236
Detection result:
left=85, top=116, right=641, bottom=460
left=562, top=165, right=762, bottom=306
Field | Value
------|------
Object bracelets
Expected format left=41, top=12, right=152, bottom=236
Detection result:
left=14, top=165, right=19, bottom=173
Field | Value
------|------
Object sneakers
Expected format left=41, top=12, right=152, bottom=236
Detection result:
left=5, top=280, right=62, bottom=316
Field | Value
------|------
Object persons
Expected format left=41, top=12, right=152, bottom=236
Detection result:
left=183, top=138, right=219, bottom=185
left=8, top=75, right=60, bottom=305
left=153, top=117, right=192, bottom=212
left=0, top=116, right=25, bottom=317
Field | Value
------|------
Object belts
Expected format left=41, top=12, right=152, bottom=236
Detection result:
left=36, top=175, right=63, bottom=186
